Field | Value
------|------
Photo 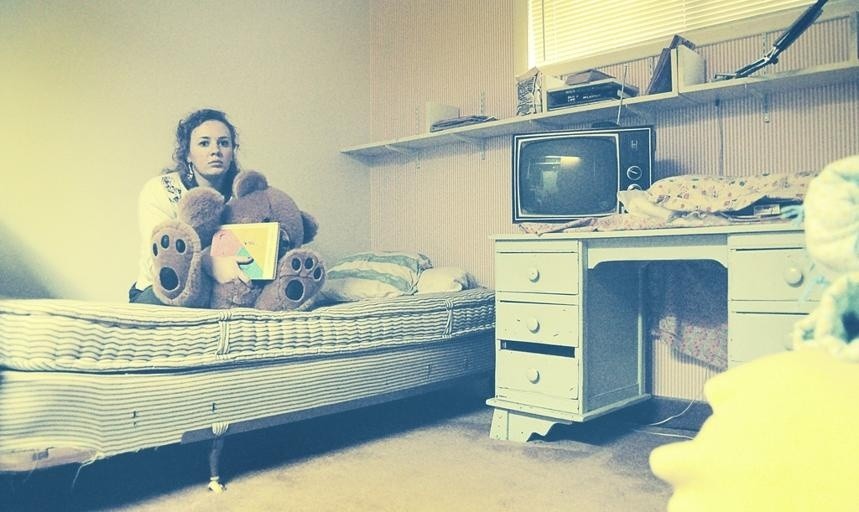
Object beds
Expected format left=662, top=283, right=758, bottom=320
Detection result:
left=0, top=288, right=494, bottom=493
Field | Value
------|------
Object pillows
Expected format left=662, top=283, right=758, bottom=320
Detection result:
left=319, top=250, right=475, bottom=304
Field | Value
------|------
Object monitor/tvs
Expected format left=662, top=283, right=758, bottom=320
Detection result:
left=512, top=123, right=656, bottom=223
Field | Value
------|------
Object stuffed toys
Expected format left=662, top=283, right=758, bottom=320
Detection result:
left=149, top=170, right=327, bottom=312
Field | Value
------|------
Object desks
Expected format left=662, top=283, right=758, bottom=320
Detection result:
left=484, top=222, right=829, bottom=447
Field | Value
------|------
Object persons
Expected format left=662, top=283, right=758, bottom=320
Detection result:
left=129, top=109, right=254, bottom=305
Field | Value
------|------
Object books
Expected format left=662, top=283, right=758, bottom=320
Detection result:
left=209, top=222, right=280, bottom=280
left=645, top=34, right=706, bottom=95
